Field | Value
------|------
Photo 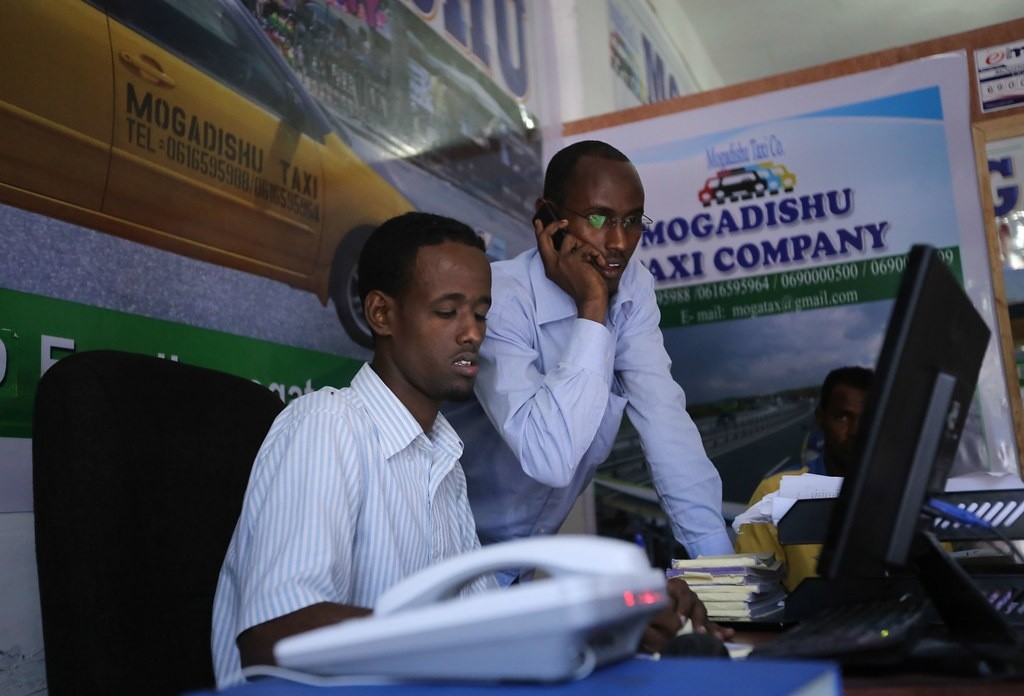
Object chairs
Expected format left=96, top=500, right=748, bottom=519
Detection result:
left=31, top=350, right=287, bottom=696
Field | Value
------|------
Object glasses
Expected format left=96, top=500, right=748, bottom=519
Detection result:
left=560, top=203, right=654, bottom=234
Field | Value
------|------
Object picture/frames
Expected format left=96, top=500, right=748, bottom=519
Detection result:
left=972, top=114, right=1024, bottom=485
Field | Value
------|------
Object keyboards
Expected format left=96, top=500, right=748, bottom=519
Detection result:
left=743, top=592, right=932, bottom=669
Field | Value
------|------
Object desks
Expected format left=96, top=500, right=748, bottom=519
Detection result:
left=714, top=625, right=1024, bottom=696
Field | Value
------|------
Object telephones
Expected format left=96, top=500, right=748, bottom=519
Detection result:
left=272, top=532, right=667, bottom=680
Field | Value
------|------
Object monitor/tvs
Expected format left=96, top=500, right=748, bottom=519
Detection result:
left=816, top=244, right=1024, bottom=680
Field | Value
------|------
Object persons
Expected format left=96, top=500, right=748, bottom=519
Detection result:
left=211, top=212, right=502, bottom=692
left=445, top=140, right=736, bottom=589
left=737, top=367, right=953, bottom=596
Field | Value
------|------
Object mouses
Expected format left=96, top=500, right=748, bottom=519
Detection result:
left=658, top=632, right=730, bottom=660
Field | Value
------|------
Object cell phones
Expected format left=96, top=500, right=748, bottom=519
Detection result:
left=532, top=203, right=568, bottom=252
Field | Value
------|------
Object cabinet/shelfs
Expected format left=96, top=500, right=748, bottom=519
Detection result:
left=933, top=487, right=1024, bottom=624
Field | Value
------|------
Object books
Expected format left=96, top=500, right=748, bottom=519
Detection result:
left=665, top=552, right=788, bottom=626
